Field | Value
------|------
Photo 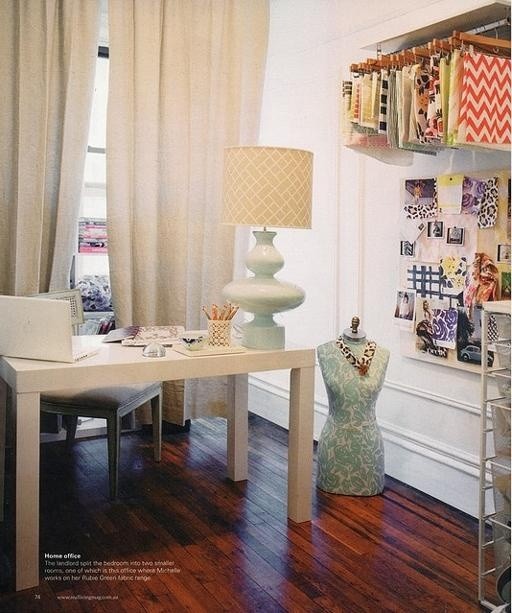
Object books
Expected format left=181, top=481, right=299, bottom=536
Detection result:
left=101, top=325, right=139, bottom=343
left=120, top=325, right=186, bottom=347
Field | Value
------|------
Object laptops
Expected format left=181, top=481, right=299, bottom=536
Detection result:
left=0, top=296, right=100, bottom=365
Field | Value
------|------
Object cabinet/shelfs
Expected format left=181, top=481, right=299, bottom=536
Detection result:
left=475, top=301, right=511, bottom=613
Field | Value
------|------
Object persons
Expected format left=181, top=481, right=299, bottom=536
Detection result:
left=398, top=294, right=410, bottom=319
left=316, top=315, right=390, bottom=496
left=413, top=182, right=423, bottom=204
left=432, top=224, right=441, bottom=237
left=422, top=299, right=434, bottom=321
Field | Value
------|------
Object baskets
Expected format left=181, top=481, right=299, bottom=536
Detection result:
left=207, top=320, right=232, bottom=349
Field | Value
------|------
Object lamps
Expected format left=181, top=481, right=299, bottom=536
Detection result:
left=219, top=146, right=316, bottom=351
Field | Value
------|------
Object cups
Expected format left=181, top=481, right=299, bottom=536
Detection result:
left=181, top=333, right=207, bottom=352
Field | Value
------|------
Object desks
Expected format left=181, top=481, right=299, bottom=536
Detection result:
left=1, top=326, right=322, bottom=593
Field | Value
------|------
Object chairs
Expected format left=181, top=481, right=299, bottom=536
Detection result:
left=20, top=289, right=164, bottom=499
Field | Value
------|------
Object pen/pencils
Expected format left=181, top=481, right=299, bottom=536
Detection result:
left=202, top=303, right=240, bottom=320
